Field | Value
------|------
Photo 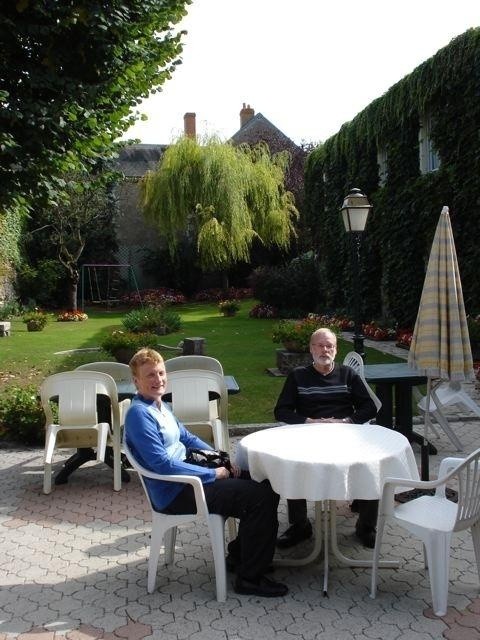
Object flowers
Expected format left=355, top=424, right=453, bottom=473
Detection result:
left=218, top=299, right=241, bottom=315
left=99, top=325, right=161, bottom=358
left=265, top=316, right=347, bottom=348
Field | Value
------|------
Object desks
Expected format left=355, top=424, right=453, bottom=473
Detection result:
left=236, top=421, right=427, bottom=599
left=49, top=364, right=242, bottom=463
left=349, top=357, right=443, bottom=458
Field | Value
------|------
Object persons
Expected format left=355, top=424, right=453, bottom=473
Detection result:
left=125, top=346, right=289, bottom=597
left=274, top=327, right=383, bottom=548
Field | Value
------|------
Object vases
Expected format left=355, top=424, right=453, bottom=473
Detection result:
left=111, top=347, right=137, bottom=362
left=224, top=311, right=235, bottom=317
left=280, top=333, right=314, bottom=351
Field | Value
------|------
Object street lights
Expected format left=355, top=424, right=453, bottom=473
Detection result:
left=339, top=186, right=375, bottom=362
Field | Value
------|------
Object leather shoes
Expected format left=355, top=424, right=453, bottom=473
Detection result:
left=224, top=554, right=240, bottom=575
left=354, top=519, right=377, bottom=549
left=275, top=517, right=314, bottom=550
left=233, top=573, right=289, bottom=598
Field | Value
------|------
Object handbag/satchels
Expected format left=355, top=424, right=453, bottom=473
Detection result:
left=181, top=446, right=234, bottom=478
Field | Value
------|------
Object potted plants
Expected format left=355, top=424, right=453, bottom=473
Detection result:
left=24, top=307, right=45, bottom=330
left=123, top=306, right=180, bottom=337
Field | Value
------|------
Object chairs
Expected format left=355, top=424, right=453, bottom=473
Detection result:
left=116, top=434, right=237, bottom=611
left=346, top=350, right=381, bottom=408
left=417, top=378, right=480, bottom=453
left=75, top=358, right=135, bottom=381
left=364, top=446, right=478, bottom=620
left=164, top=368, right=234, bottom=460
left=36, top=362, right=124, bottom=499
left=159, top=353, right=227, bottom=375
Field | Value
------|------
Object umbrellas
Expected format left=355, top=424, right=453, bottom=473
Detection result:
left=393, top=204, right=474, bottom=482
